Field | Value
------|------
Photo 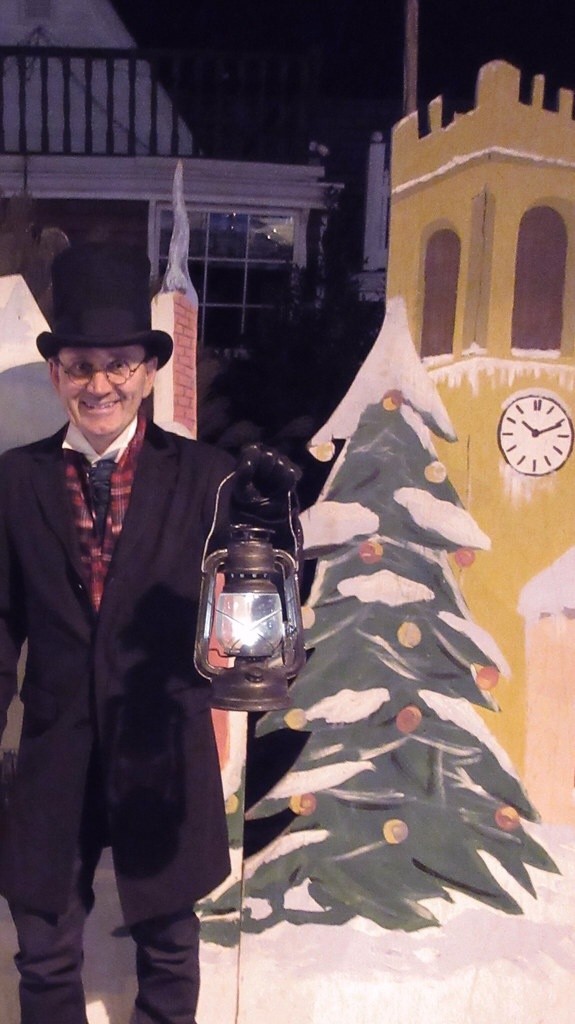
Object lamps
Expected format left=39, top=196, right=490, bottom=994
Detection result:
left=194, top=465, right=306, bottom=710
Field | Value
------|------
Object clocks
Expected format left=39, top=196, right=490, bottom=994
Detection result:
left=498, top=394, right=574, bottom=476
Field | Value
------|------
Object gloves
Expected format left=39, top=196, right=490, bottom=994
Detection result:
left=231, top=443, right=302, bottom=519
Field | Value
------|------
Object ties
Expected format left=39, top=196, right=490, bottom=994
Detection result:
left=91, top=458, right=116, bottom=543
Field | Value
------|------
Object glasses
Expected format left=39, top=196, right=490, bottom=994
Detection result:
left=56, top=357, right=147, bottom=386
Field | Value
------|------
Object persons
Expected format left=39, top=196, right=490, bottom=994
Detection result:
left=0, top=241, right=301, bottom=1024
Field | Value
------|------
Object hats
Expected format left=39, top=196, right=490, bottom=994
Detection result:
left=36, top=240, right=173, bottom=371
